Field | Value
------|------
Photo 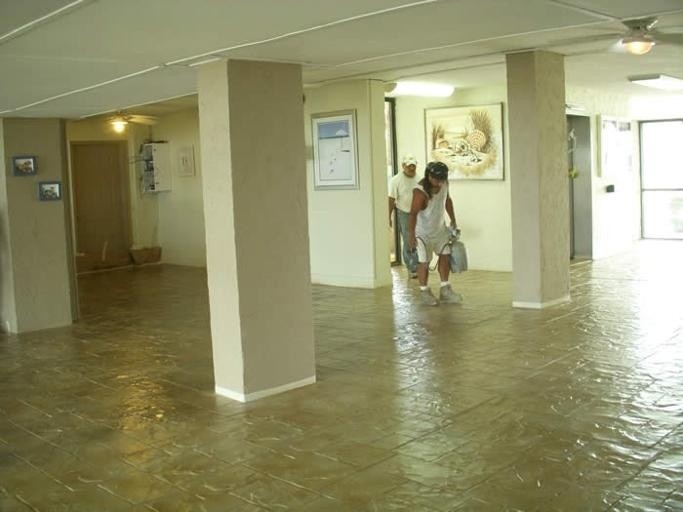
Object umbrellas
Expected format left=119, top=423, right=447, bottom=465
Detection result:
left=334, top=129, right=348, bottom=150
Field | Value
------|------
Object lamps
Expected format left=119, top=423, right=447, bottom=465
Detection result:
left=618, top=32, right=658, bottom=56
left=107, top=117, right=129, bottom=133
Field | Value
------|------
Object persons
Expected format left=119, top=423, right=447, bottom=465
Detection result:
left=389, top=155, right=422, bottom=279
left=408, top=162, right=463, bottom=306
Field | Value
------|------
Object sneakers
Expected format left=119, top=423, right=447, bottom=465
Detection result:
left=421, top=284, right=463, bottom=305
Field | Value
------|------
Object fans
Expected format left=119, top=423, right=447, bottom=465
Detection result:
left=544, top=14, right=683, bottom=55
left=90, top=109, right=160, bottom=127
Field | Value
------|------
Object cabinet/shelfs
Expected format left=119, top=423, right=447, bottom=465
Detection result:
left=140, top=141, right=171, bottom=192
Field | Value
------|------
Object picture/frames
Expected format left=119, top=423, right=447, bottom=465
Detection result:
left=10, top=155, right=37, bottom=178
left=309, top=109, right=360, bottom=193
left=37, top=180, right=61, bottom=205
left=421, top=100, right=505, bottom=183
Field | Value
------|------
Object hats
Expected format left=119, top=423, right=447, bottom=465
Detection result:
left=401, top=157, right=418, bottom=167
